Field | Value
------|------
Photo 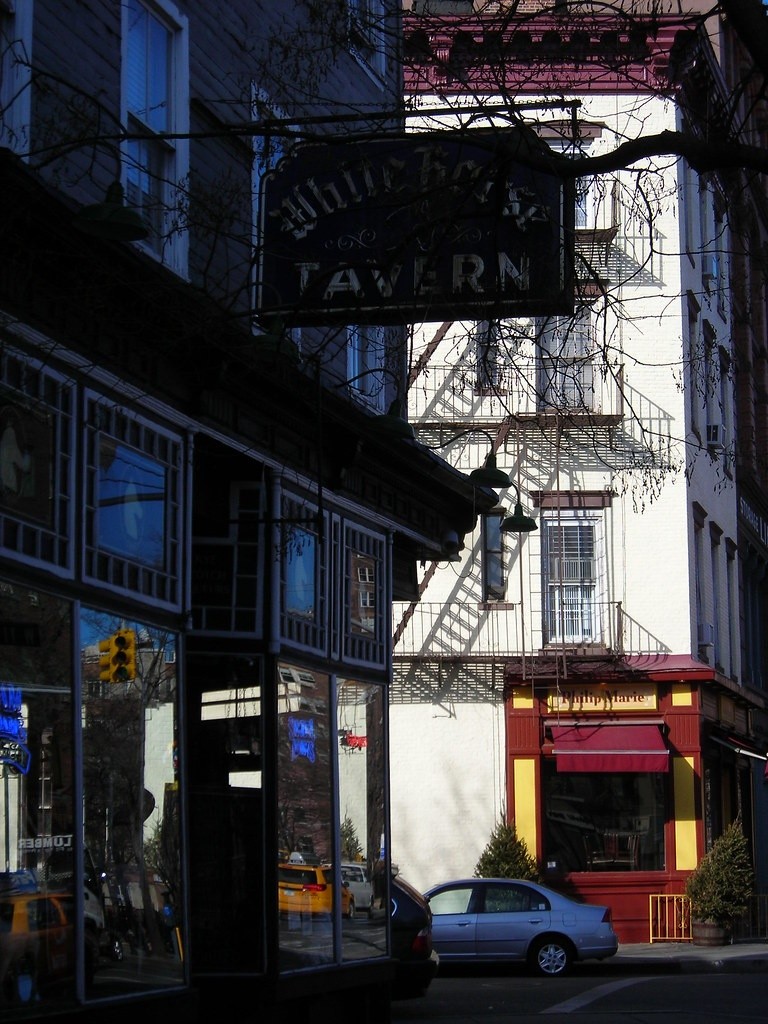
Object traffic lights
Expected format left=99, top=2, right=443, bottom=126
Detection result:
left=109, top=632, right=136, bottom=682
left=98, top=634, right=113, bottom=683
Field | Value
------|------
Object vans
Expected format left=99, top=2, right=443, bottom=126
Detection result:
left=323, top=862, right=374, bottom=920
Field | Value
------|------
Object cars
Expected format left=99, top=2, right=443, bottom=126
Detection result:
left=0, top=869, right=74, bottom=996
left=419, top=877, right=619, bottom=978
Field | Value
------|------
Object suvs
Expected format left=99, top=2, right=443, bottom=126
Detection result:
left=278, top=851, right=355, bottom=922
left=389, top=861, right=441, bottom=1000
